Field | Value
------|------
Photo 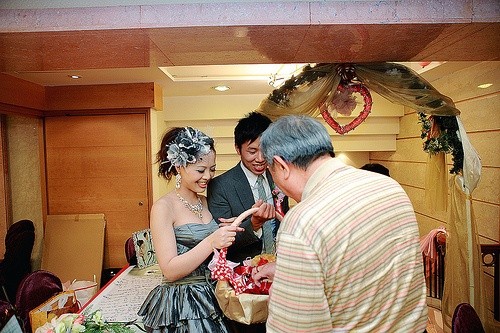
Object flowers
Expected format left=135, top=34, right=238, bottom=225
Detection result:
left=272, top=186, right=288, bottom=201
left=159, top=144, right=190, bottom=172
left=35, top=310, right=135, bottom=333
left=331, top=89, right=358, bottom=116
left=177, top=126, right=212, bottom=156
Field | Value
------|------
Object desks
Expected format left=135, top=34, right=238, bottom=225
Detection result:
left=75, top=261, right=163, bottom=333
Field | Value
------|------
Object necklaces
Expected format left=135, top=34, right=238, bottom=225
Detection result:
left=175, top=189, right=203, bottom=218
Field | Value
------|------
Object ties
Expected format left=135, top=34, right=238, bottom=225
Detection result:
left=257, top=174, right=275, bottom=255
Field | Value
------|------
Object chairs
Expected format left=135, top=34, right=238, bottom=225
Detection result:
left=19, top=270, right=63, bottom=333
left=0, top=219, right=35, bottom=303
left=452, top=303, right=485, bottom=333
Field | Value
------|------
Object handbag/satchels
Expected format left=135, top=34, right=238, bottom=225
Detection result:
left=29, top=290, right=82, bottom=333
left=62, top=275, right=97, bottom=310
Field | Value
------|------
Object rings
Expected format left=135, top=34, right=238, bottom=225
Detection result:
left=231, top=237, right=232, bottom=242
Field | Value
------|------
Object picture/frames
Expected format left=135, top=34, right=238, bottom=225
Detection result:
left=132, top=228, right=158, bottom=270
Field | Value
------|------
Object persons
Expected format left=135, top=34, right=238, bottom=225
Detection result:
left=261, top=117, right=428, bottom=333
left=138, top=127, right=245, bottom=333
left=208, top=112, right=289, bottom=333
